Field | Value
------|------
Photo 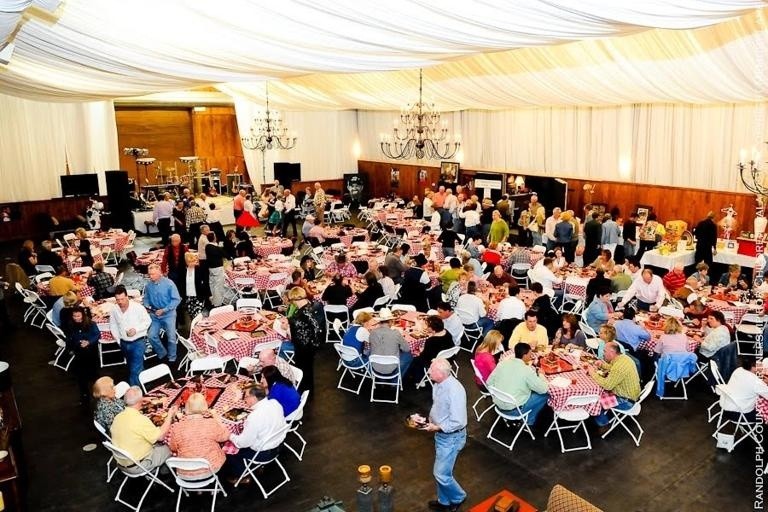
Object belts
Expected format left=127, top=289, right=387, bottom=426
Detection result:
left=437, top=427, right=465, bottom=433
left=495, top=405, right=522, bottom=413
left=615, top=395, right=635, bottom=405
left=117, top=459, right=143, bottom=469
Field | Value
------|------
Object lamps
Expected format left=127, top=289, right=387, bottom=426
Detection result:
left=737, top=139, right=767, bottom=199
left=380, top=67, right=461, bottom=160
left=242, top=78, right=298, bottom=152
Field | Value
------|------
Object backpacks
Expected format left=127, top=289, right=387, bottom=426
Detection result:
left=435, top=208, right=453, bottom=230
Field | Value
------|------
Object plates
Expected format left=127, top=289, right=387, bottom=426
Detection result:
left=251, top=330, right=267, bottom=338
left=199, top=328, right=219, bottom=336
left=391, top=310, right=408, bottom=319
left=140, top=374, right=251, bottom=427
left=404, top=415, right=432, bottom=430
left=234, top=261, right=281, bottom=275
left=417, top=315, right=430, bottom=327
left=265, top=314, right=276, bottom=320
left=686, top=330, right=705, bottom=338
left=410, top=330, right=429, bottom=339
left=580, top=356, right=598, bottom=363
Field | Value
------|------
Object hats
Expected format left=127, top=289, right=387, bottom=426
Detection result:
left=300, top=255, right=314, bottom=266
left=306, top=214, right=315, bottom=220
left=431, top=202, right=443, bottom=208
left=378, top=307, right=398, bottom=322
left=413, top=253, right=427, bottom=266
left=335, top=253, right=348, bottom=264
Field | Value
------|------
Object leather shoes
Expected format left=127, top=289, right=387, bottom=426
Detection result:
left=226, top=476, right=251, bottom=484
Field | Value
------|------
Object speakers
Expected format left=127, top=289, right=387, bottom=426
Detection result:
left=105, top=170, right=131, bottom=232
left=274, top=161, right=302, bottom=183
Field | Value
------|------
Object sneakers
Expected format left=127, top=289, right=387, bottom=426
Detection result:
left=503, top=417, right=517, bottom=430
left=415, top=382, right=425, bottom=387
left=449, top=493, right=468, bottom=512
left=428, top=500, right=450, bottom=512
left=523, top=426, right=536, bottom=436
left=591, top=423, right=612, bottom=436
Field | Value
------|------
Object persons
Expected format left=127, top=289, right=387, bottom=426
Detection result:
left=420, top=358, right=469, bottom=512
left=165, top=392, right=229, bottom=496
left=227, top=385, right=287, bottom=485
left=8, top=180, right=767, bottom=452
left=109, top=385, right=177, bottom=488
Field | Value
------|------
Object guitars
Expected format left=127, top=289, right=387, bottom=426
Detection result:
left=231, top=164, right=239, bottom=193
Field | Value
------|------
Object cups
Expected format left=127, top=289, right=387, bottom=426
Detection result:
left=572, top=377, right=576, bottom=384
left=584, top=362, right=588, bottom=369
